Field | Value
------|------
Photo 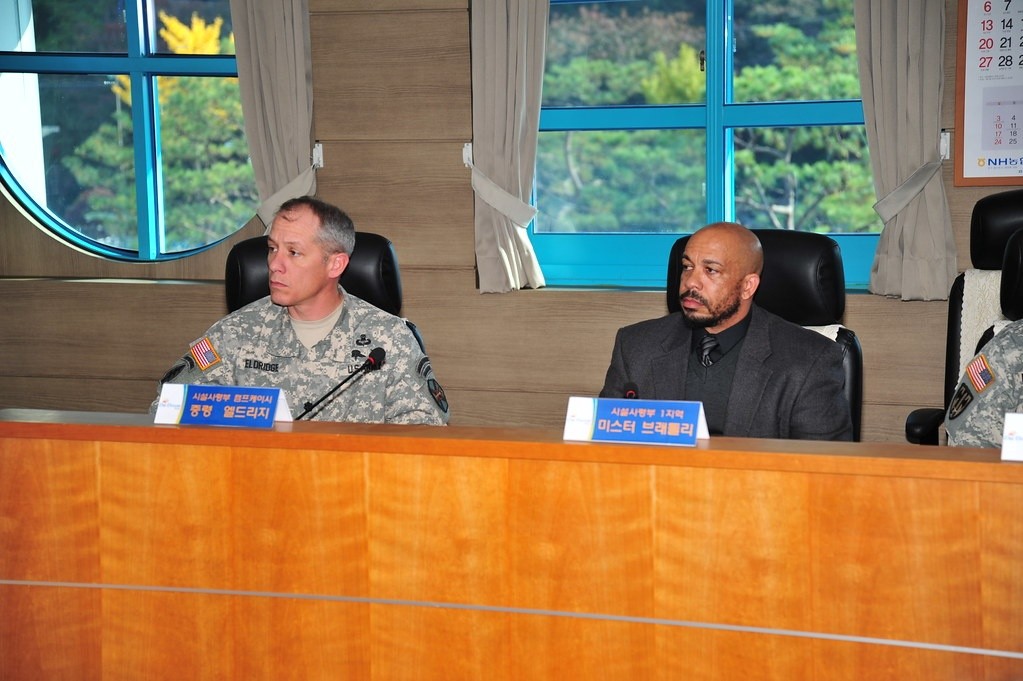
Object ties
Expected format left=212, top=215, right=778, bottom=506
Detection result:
left=702, top=336, right=718, bottom=366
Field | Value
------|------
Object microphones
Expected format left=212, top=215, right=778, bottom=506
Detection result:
left=293, top=347, right=387, bottom=420
left=624, top=382, right=638, bottom=399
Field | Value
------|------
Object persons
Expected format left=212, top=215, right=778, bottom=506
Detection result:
left=595, top=220, right=855, bottom=443
left=944, top=318, right=1022, bottom=451
left=146, top=196, right=451, bottom=426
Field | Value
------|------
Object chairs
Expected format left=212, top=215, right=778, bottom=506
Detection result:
left=225, top=232, right=402, bottom=316
left=664, top=229, right=865, bottom=441
left=903, top=188, right=1023, bottom=446
